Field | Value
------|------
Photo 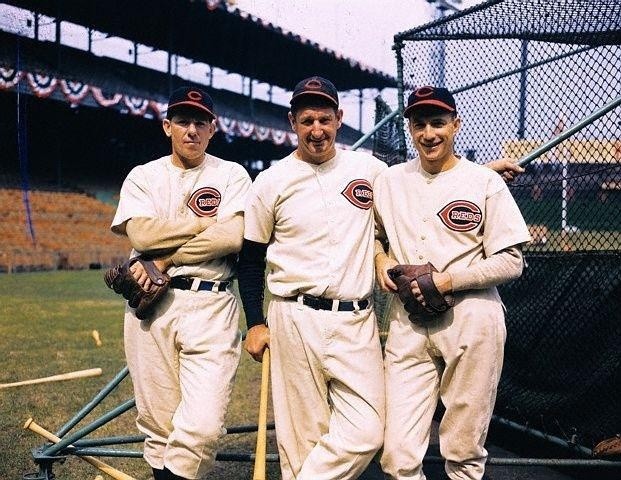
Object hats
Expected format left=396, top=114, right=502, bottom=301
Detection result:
left=289, top=77, right=339, bottom=107
left=403, top=86, right=456, bottom=117
left=168, top=87, right=217, bottom=121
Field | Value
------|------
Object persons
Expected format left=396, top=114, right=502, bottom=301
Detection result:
left=101, top=82, right=258, bottom=478
left=237, top=75, right=531, bottom=480
left=367, top=86, right=535, bottom=480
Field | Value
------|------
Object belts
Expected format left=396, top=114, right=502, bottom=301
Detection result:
left=287, top=292, right=368, bottom=311
left=170, top=275, right=228, bottom=291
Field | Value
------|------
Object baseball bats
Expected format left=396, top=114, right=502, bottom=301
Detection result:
left=0, top=367, right=102, bottom=389
left=23, top=417, right=137, bottom=480
left=253, top=348, right=270, bottom=480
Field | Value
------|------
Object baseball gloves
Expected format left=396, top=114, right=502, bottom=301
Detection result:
left=104, top=255, right=170, bottom=320
left=592, top=435, right=619, bottom=458
left=387, top=262, right=454, bottom=327
